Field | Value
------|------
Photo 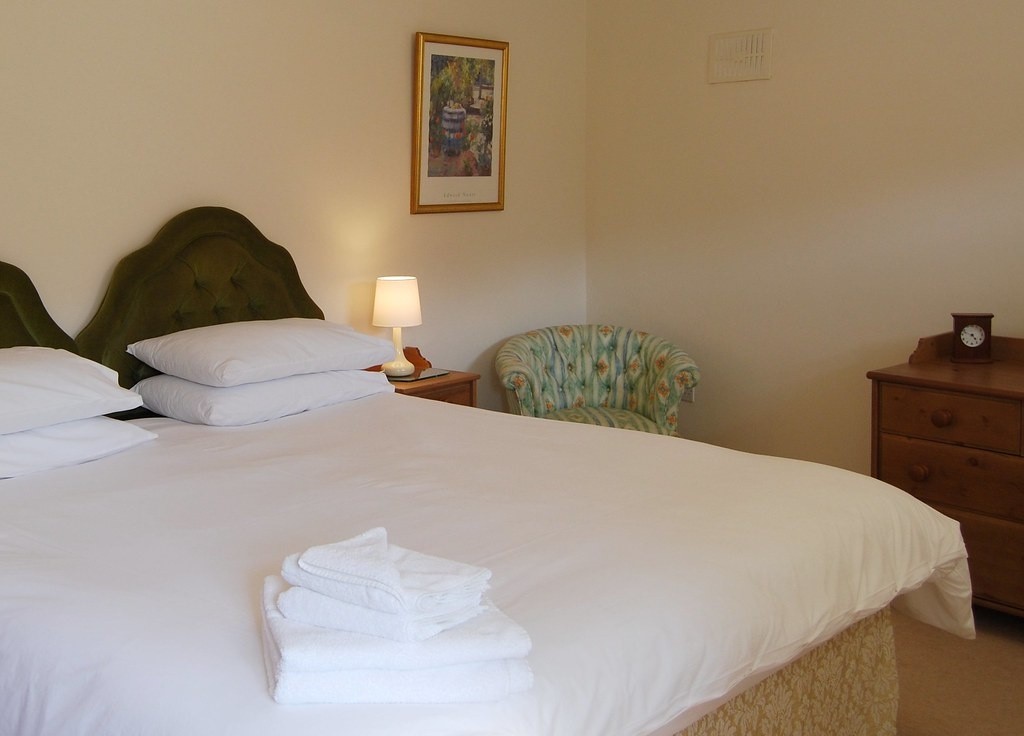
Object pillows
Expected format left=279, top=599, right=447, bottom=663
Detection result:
left=129, top=369, right=396, bottom=426
left=0, top=346, right=143, bottom=436
left=126, top=318, right=396, bottom=388
left=0, top=416, right=158, bottom=479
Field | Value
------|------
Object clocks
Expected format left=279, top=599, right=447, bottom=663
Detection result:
left=951, top=312, right=995, bottom=365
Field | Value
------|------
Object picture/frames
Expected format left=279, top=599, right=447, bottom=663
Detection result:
left=410, top=32, right=509, bottom=214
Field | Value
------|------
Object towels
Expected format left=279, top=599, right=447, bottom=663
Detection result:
left=257, top=525, right=535, bottom=703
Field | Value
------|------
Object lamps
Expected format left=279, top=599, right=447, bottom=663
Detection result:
left=372, top=276, right=422, bottom=376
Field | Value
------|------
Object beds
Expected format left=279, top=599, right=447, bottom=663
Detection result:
left=1, top=205, right=978, bottom=736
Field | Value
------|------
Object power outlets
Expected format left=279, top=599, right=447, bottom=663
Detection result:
left=681, top=386, right=694, bottom=402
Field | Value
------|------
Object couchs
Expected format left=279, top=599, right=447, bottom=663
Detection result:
left=494, top=324, right=701, bottom=438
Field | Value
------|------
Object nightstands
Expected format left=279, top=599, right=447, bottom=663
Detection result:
left=362, top=346, right=480, bottom=408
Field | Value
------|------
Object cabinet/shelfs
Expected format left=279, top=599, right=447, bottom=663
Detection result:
left=866, top=330, right=1023, bottom=617
